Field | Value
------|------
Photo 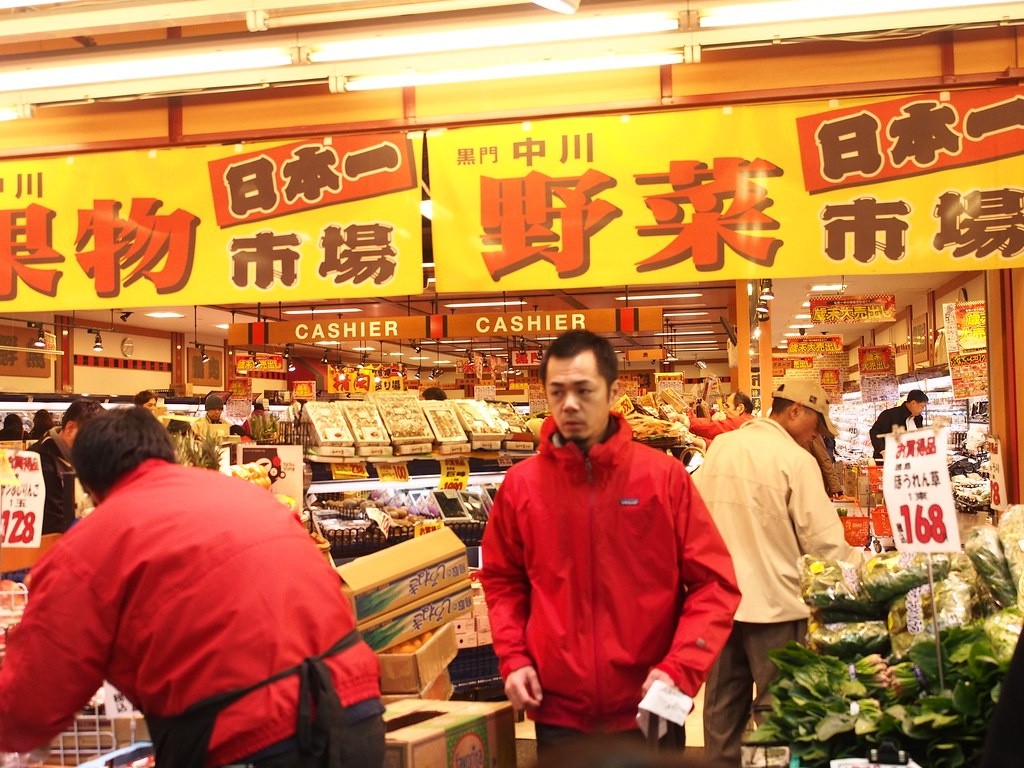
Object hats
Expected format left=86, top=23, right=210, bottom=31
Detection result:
left=772, top=378, right=840, bottom=436
left=205, top=395, right=224, bottom=410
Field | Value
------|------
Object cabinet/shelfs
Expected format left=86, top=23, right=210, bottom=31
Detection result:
left=836, top=368, right=988, bottom=501
left=280, top=421, right=541, bottom=568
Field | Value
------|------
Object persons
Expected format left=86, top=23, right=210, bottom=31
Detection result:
left=692, top=379, right=867, bottom=756
left=685, top=392, right=844, bottom=500
left=869, top=390, right=929, bottom=466
left=422, top=387, right=448, bottom=400
left=0, top=391, right=277, bottom=588
left=478, top=329, right=743, bottom=757
left=0, top=408, right=387, bottom=768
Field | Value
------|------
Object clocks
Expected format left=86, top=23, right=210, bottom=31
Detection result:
left=120, top=337, right=133, bottom=357
left=957, top=288, right=967, bottom=310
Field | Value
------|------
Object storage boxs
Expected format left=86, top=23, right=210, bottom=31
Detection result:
left=837, top=464, right=883, bottom=507
left=0, top=394, right=528, bottom=768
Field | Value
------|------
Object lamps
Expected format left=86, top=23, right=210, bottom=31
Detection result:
left=752, top=279, right=774, bottom=338
left=0, top=0, right=1024, bottom=121
left=26, top=312, right=542, bottom=382
left=694, top=353, right=706, bottom=370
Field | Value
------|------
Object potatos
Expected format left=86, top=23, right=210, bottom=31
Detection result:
left=325, top=497, right=428, bottom=527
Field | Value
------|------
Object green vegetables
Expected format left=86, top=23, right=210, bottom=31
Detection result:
left=749, top=505, right=1023, bottom=768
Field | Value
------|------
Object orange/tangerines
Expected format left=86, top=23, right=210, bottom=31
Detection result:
left=381, top=632, right=433, bottom=654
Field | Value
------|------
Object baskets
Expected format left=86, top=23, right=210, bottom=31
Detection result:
left=320, top=485, right=511, bottom=555
left=840, top=516, right=870, bottom=546
left=871, top=505, right=893, bottom=536
left=868, top=465, right=884, bottom=493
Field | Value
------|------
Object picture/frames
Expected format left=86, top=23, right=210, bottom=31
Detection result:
left=911, top=314, right=929, bottom=364
